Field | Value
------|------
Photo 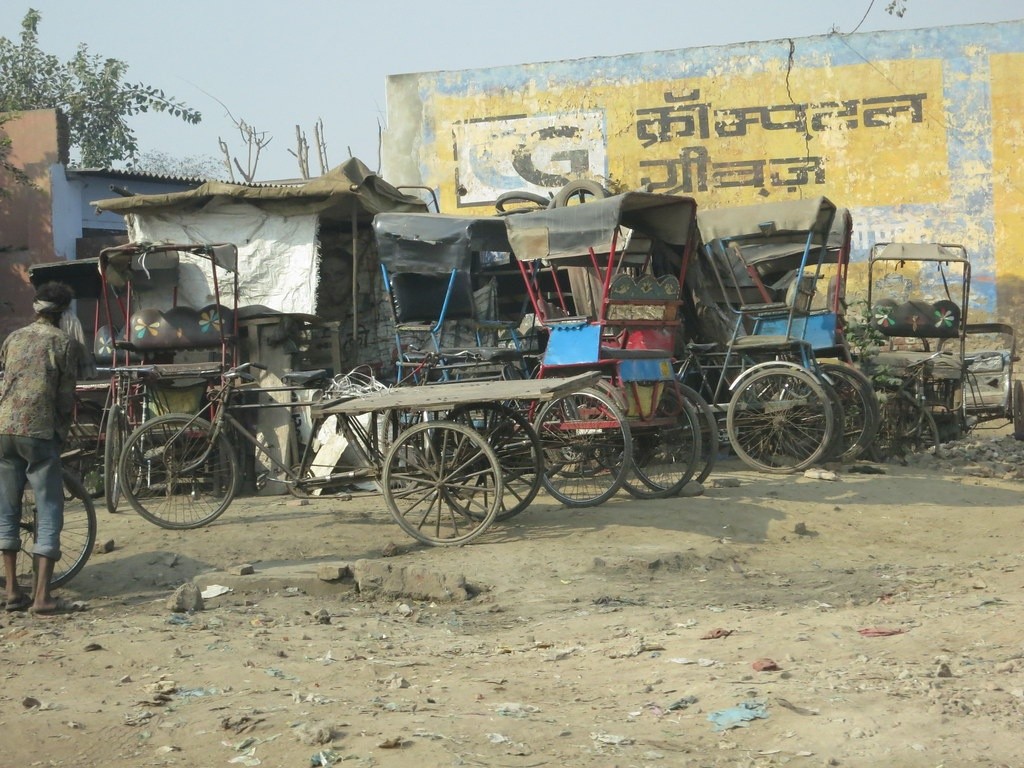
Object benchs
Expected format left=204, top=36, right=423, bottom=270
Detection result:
left=389, top=271, right=523, bottom=365
left=599, top=271, right=682, bottom=361
left=952, top=351, right=1008, bottom=406
left=115, top=304, right=234, bottom=377
left=726, top=272, right=815, bottom=355
left=76, top=325, right=174, bottom=388
left=862, top=298, right=962, bottom=380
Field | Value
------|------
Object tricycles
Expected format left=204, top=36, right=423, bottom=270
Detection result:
left=0, top=187, right=1023, bottom=600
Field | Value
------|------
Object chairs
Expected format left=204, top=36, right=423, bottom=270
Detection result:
left=758, top=273, right=845, bottom=358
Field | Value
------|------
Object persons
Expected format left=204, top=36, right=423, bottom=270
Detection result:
left=0, top=282, right=84, bottom=616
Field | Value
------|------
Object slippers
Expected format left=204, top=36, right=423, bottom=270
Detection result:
left=40, top=596, right=75, bottom=615
left=5, top=594, right=32, bottom=609
